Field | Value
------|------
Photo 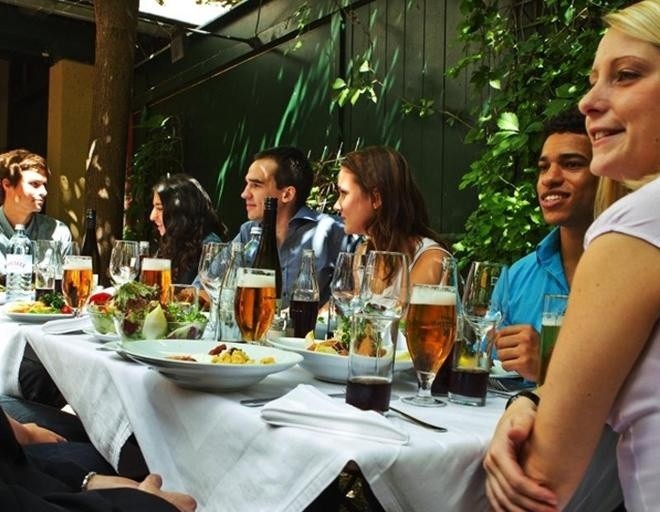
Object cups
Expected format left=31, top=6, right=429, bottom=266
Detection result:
left=448, top=317, right=496, bottom=406
left=35, top=241, right=57, bottom=301
left=537, top=295, right=570, bottom=388
left=61, top=256, right=93, bottom=317
left=345, top=314, right=398, bottom=414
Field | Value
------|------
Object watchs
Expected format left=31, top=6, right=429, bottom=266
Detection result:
left=504, top=391, right=540, bottom=409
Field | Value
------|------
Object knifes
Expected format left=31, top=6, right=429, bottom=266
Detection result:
left=243, top=390, right=345, bottom=405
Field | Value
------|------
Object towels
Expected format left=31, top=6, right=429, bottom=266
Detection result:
left=43, top=316, right=89, bottom=334
left=261, top=384, right=409, bottom=445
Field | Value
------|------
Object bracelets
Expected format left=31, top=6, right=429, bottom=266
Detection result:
left=80, top=470, right=97, bottom=491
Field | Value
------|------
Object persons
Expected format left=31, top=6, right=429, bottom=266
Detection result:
left=0, top=407, right=199, bottom=507
left=197, top=148, right=359, bottom=331
left=137, top=175, right=229, bottom=301
left=331, top=145, right=464, bottom=368
left=480, top=0, right=658, bottom=508
left=483, top=107, right=614, bottom=386
left=0, top=151, right=71, bottom=286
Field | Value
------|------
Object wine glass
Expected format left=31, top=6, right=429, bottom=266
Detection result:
left=139, top=258, right=171, bottom=306
left=109, top=241, right=142, bottom=288
left=359, top=251, right=410, bottom=339
left=235, top=268, right=276, bottom=344
left=331, top=252, right=369, bottom=324
left=167, top=284, right=199, bottom=331
left=200, top=243, right=234, bottom=338
left=463, top=260, right=508, bottom=370
left=405, top=285, right=457, bottom=407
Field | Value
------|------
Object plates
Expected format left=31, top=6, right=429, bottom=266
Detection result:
left=486, top=358, right=522, bottom=379
left=7, top=307, right=80, bottom=322
left=106, top=339, right=303, bottom=392
left=270, top=337, right=414, bottom=384
left=82, top=317, right=129, bottom=342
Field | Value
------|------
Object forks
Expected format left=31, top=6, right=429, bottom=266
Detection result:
left=387, top=406, right=449, bottom=432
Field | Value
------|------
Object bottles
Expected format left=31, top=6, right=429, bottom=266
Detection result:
left=289, top=250, right=320, bottom=336
left=250, top=196, right=281, bottom=318
left=5, top=224, right=35, bottom=307
left=138, top=239, right=152, bottom=278
left=79, top=208, right=102, bottom=282
left=430, top=257, right=464, bottom=397
